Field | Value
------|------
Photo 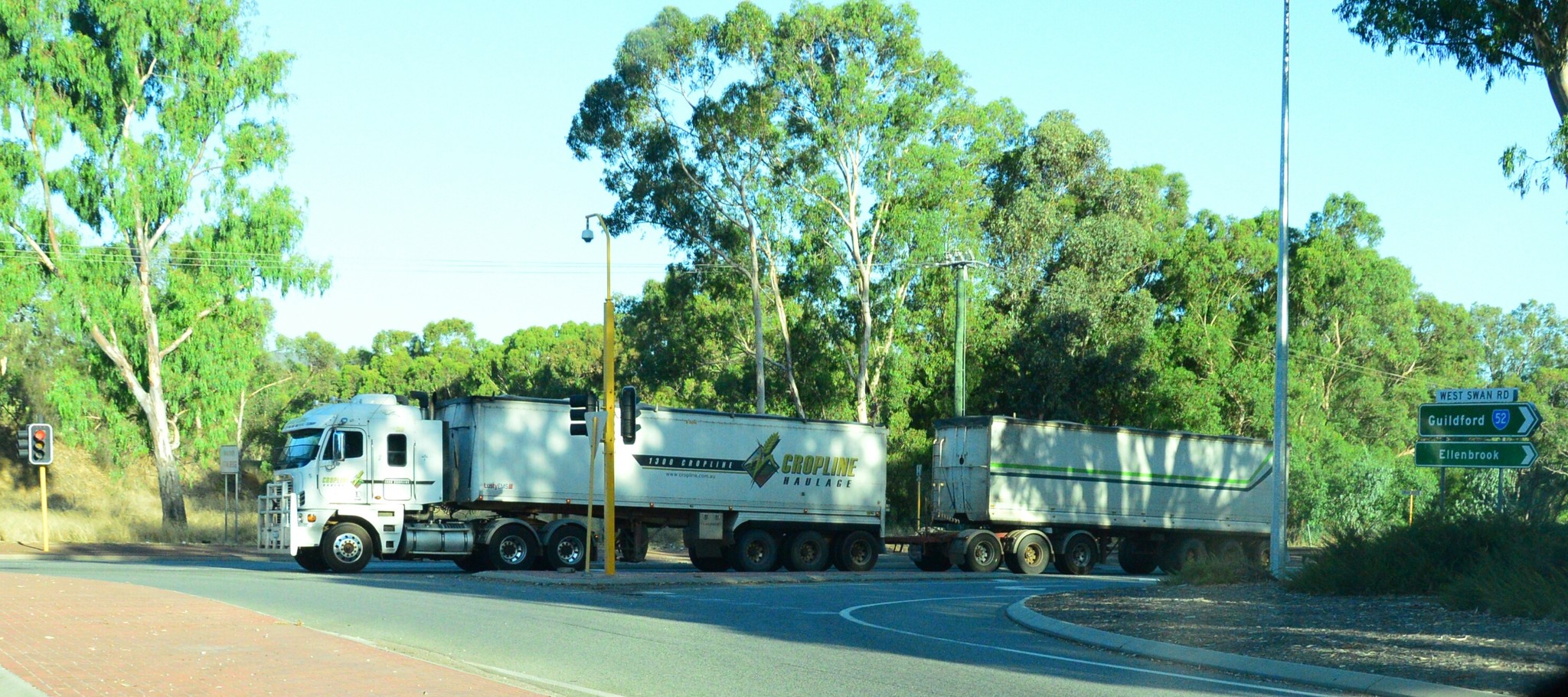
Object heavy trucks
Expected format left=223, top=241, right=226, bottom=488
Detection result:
left=255, top=389, right=889, bottom=574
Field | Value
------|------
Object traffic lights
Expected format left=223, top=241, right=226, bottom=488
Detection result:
left=15, top=424, right=31, bottom=465
left=618, top=386, right=642, bottom=445
left=569, top=391, right=597, bottom=437
left=28, top=423, right=53, bottom=465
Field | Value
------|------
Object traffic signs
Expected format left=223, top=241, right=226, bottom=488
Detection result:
left=1414, top=440, right=1538, bottom=469
left=1417, top=402, right=1544, bottom=439
left=1401, top=490, right=1422, bottom=496
